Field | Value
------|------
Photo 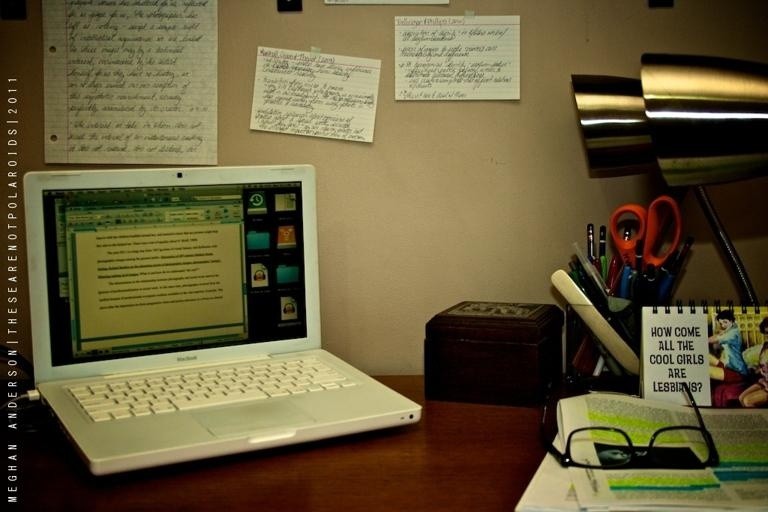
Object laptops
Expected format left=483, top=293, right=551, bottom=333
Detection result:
left=23, top=164, right=423, bottom=476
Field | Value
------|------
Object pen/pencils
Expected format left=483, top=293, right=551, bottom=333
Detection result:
left=568, top=224, right=694, bottom=378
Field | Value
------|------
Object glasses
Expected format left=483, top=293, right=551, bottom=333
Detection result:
left=540, top=381, right=719, bottom=468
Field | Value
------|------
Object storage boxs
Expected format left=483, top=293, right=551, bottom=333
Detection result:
left=418, top=299, right=566, bottom=409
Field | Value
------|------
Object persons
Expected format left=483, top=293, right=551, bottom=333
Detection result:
left=737, top=316, right=768, bottom=408
left=707, top=309, right=748, bottom=383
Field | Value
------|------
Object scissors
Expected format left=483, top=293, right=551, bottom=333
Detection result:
left=610, top=196, right=683, bottom=272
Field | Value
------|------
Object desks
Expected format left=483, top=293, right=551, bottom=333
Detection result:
left=0, top=374, right=767, bottom=512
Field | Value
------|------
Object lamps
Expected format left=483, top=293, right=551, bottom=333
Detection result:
left=641, top=52, right=768, bottom=190
left=568, top=72, right=763, bottom=303
